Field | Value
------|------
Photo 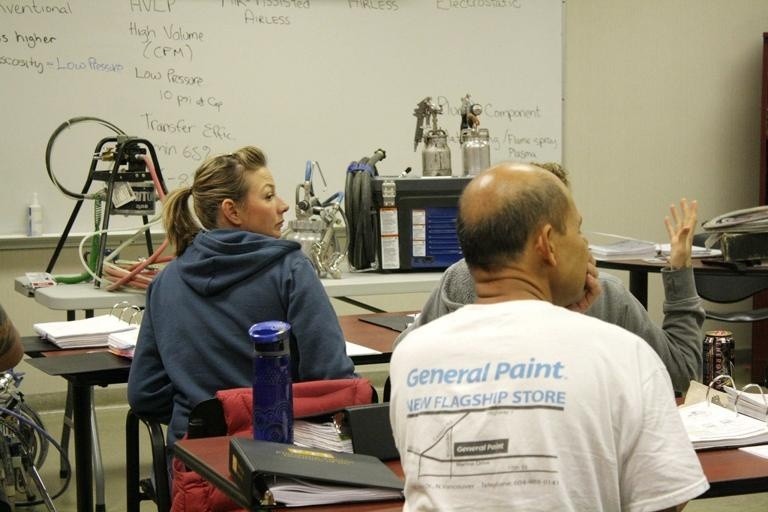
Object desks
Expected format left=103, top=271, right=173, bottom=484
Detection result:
left=14, top=232, right=768, bottom=512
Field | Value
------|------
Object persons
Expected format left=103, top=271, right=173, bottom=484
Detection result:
left=411, top=152, right=709, bottom=406
left=123, top=144, right=359, bottom=511
left=381, top=161, right=710, bottom=512
left=0, top=302, right=51, bottom=511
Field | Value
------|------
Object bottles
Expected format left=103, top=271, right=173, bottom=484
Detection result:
left=28, top=193, right=43, bottom=236
left=250, top=320, right=292, bottom=443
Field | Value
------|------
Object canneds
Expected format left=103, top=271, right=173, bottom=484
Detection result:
left=703, top=330, right=736, bottom=391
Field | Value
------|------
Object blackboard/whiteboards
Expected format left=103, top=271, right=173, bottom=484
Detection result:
left=0, top=0, right=563, bottom=241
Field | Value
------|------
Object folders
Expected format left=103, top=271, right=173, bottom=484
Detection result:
left=229, top=437, right=405, bottom=509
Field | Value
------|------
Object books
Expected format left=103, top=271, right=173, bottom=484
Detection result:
left=581, top=225, right=723, bottom=265
left=33, top=300, right=141, bottom=351
left=224, top=427, right=407, bottom=508
left=108, top=324, right=142, bottom=361
left=701, top=200, right=768, bottom=253
left=672, top=374, right=768, bottom=453
left=292, top=400, right=402, bottom=461
